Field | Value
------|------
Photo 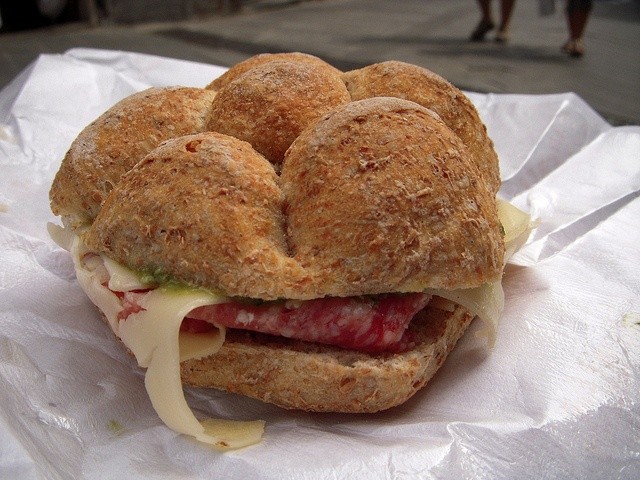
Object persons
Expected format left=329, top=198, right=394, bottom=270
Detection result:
left=468, top=0, right=516, bottom=42
left=559, top=0, right=596, bottom=57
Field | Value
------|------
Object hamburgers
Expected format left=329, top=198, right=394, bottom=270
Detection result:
left=47, top=51, right=532, bottom=451
